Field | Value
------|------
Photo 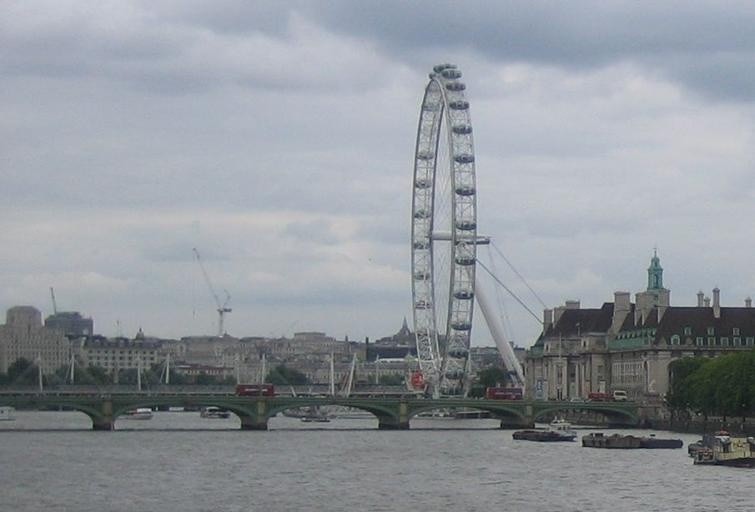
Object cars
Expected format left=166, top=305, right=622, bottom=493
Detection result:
left=568, top=396, right=585, bottom=403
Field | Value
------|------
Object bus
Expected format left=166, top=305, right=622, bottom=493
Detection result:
left=485, top=386, right=523, bottom=400
left=236, top=384, right=273, bottom=396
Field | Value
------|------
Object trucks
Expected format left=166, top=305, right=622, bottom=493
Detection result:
left=588, top=388, right=627, bottom=402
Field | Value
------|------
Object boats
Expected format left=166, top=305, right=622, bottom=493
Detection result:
left=0, top=405, right=19, bottom=431
left=198, top=405, right=231, bottom=418
left=498, top=417, right=755, bottom=465
left=116, top=408, right=154, bottom=422
left=268, top=405, right=331, bottom=424
